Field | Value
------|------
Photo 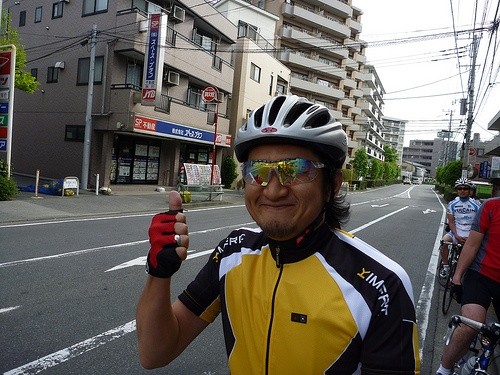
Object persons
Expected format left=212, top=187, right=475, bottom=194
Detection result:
left=436, top=197, right=500, bottom=375
left=136, top=94, right=421, bottom=375
left=440, top=177, right=484, bottom=279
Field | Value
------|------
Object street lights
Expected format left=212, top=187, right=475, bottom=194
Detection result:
left=442, top=129, right=472, bottom=179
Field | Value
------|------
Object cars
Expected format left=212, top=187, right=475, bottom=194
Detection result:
left=403, top=178, right=411, bottom=185
left=413, top=178, right=420, bottom=184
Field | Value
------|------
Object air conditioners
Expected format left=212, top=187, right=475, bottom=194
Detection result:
left=165, top=71, right=180, bottom=86
left=170, top=5, right=185, bottom=23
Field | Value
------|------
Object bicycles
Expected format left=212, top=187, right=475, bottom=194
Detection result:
left=445, top=295, right=499, bottom=375
left=436, top=239, right=463, bottom=315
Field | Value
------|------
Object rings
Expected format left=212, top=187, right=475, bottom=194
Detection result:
left=174, top=234, right=181, bottom=246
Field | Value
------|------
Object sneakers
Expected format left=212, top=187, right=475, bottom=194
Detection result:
left=440, top=265, right=450, bottom=277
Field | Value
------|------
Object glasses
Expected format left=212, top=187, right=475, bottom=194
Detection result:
left=457, top=187, right=469, bottom=190
left=240, top=157, right=332, bottom=186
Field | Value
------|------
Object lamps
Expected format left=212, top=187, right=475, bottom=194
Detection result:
left=55, top=61, right=64, bottom=69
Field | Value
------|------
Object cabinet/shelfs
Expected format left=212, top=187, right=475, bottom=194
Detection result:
left=178, top=184, right=225, bottom=202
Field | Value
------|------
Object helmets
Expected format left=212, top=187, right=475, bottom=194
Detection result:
left=470, top=183, right=477, bottom=190
left=233, top=95, right=348, bottom=164
left=455, top=177, right=471, bottom=188
